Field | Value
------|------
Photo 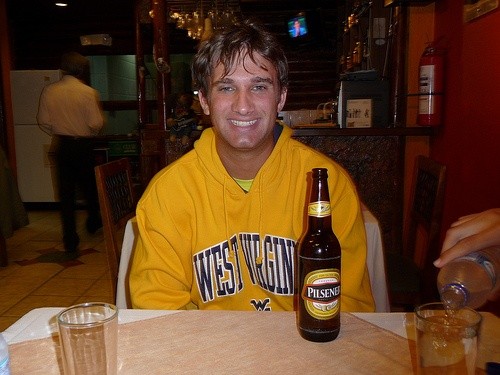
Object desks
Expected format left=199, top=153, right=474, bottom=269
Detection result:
left=0, top=306, right=500, bottom=375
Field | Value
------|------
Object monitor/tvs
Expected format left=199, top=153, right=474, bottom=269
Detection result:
left=286, top=16, right=309, bottom=38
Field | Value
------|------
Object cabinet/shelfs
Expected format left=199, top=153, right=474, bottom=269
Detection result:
left=339, top=0, right=392, bottom=82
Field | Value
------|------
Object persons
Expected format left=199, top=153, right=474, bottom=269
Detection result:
left=291, top=17, right=307, bottom=38
left=130, top=19, right=377, bottom=312
left=433, top=209, right=500, bottom=300
left=38, top=53, right=105, bottom=260
left=168, top=91, right=194, bottom=144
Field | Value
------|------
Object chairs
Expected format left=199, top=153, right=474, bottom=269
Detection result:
left=95, top=156, right=137, bottom=307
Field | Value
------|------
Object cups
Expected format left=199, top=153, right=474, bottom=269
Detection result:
left=414, top=302, right=483, bottom=375
left=56, top=302, right=119, bottom=375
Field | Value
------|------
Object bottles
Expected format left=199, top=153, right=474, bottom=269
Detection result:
left=0, top=333, right=10, bottom=375
left=294, top=168, right=340, bottom=343
left=436, top=245, right=500, bottom=311
